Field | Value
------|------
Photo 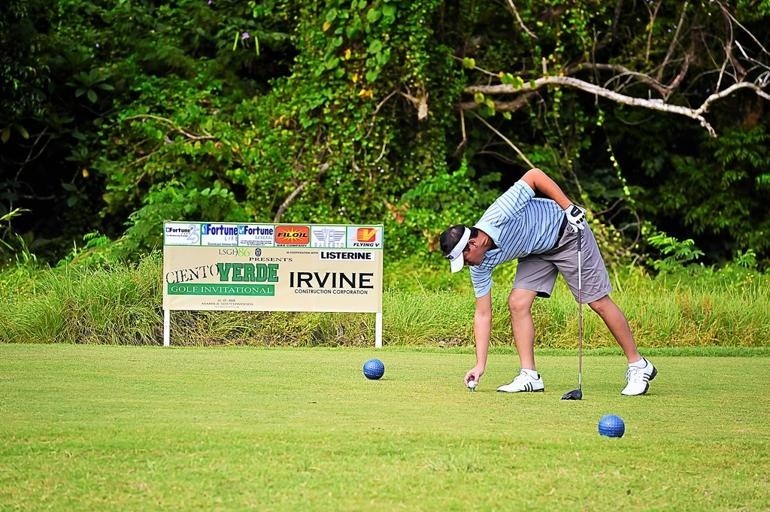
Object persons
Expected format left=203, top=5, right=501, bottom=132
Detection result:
left=439, top=168, right=659, bottom=397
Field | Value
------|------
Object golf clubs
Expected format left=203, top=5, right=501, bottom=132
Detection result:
left=561, top=228, right=582, bottom=401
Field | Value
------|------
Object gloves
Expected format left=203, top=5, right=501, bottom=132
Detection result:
left=564, top=202, right=592, bottom=234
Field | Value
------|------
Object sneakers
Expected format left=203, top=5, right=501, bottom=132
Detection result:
left=621, top=355, right=658, bottom=397
left=498, top=369, right=544, bottom=394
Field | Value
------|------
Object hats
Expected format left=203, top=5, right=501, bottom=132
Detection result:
left=444, top=225, right=472, bottom=274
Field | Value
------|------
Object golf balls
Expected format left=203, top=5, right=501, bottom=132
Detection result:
left=468, top=380, right=476, bottom=388
